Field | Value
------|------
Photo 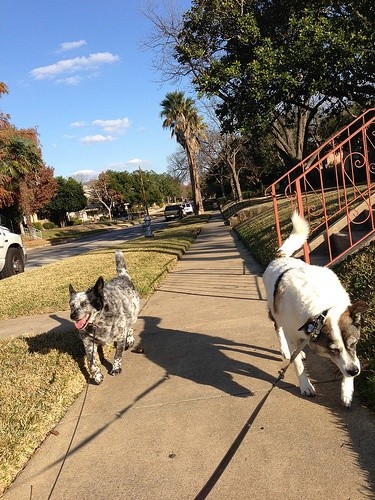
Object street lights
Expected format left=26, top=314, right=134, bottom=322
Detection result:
left=141, top=179, right=150, bottom=216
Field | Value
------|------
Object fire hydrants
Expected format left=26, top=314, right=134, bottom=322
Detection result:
left=142, top=215, right=154, bottom=237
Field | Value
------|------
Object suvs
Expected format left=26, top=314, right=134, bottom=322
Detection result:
left=182, top=203, right=193, bottom=215
left=164, top=204, right=184, bottom=221
left=0, top=224, right=28, bottom=280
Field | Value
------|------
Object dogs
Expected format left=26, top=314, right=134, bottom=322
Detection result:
left=261, top=208, right=368, bottom=409
left=68, top=251, right=139, bottom=384
